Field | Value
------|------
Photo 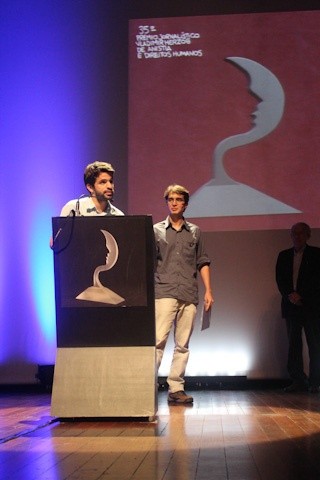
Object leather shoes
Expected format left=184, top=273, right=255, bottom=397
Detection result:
left=284, top=381, right=320, bottom=394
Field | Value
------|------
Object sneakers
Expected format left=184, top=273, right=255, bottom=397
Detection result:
left=168, top=389, right=195, bottom=407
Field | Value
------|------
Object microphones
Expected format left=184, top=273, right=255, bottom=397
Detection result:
left=76, top=194, right=96, bottom=216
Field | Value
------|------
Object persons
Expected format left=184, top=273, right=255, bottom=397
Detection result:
left=153, top=184, right=214, bottom=404
left=60, top=161, right=125, bottom=217
left=275, top=222, right=320, bottom=394
